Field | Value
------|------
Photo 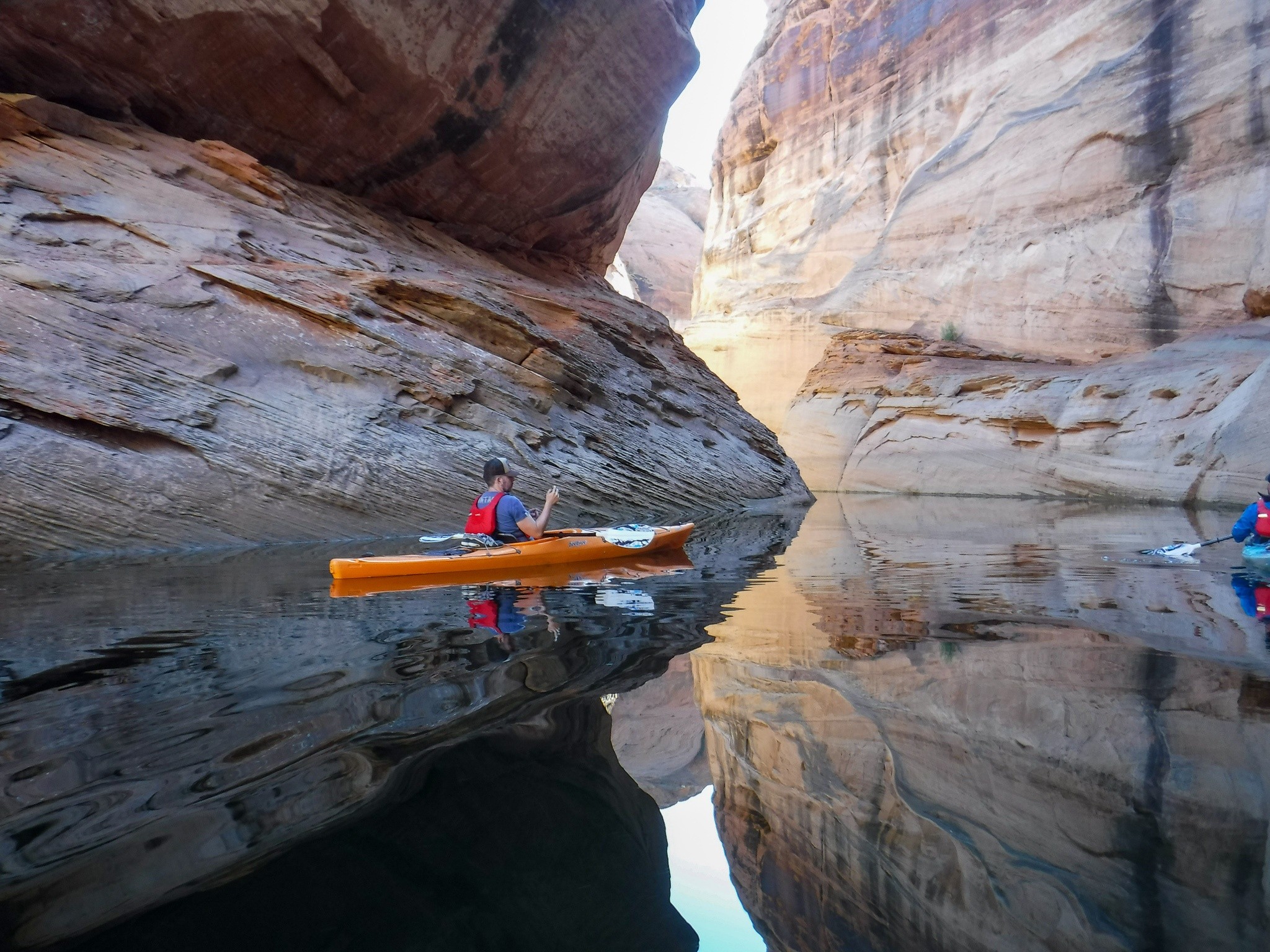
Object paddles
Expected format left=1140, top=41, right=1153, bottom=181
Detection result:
left=418, top=522, right=655, bottom=550
left=1149, top=535, right=1233, bottom=557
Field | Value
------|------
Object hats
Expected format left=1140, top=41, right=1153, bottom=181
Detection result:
left=1265, top=473, right=1270, bottom=483
left=483, top=457, right=519, bottom=477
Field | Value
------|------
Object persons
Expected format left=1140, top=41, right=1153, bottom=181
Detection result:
left=1231, top=573, right=1270, bottom=650
left=465, top=457, right=559, bottom=541
left=1232, top=474, right=1270, bottom=544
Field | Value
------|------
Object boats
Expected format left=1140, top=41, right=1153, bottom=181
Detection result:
left=329, top=522, right=695, bottom=580
left=1240, top=544, right=1270, bottom=572
left=329, top=547, right=695, bottom=598
